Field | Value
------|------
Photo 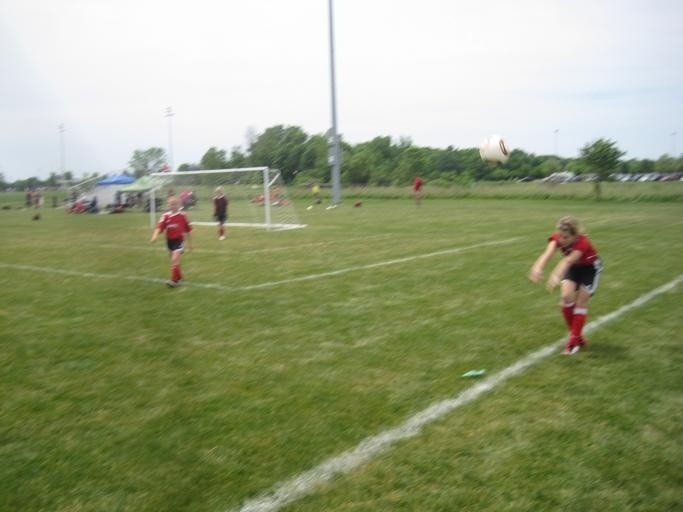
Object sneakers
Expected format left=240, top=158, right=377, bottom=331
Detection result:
left=218, top=235, right=226, bottom=241
left=165, top=275, right=184, bottom=288
left=563, top=335, right=587, bottom=353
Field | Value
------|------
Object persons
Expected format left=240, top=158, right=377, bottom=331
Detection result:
left=112, top=189, right=120, bottom=208
left=311, top=181, right=321, bottom=207
left=119, top=192, right=130, bottom=209
left=411, top=175, right=424, bottom=210
left=23, top=187, right=97, bottom=214
left=523, top=215, right=603, bottom=355
left=150, top=195, right=194, bottom=290
left=211, top=184, right=229, bottom=242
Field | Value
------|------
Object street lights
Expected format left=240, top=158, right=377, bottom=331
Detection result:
left=163, top=105, right=176, bottom=169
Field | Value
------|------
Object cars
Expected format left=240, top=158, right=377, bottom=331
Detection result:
left=617, top=171, right=683, bottom=183
